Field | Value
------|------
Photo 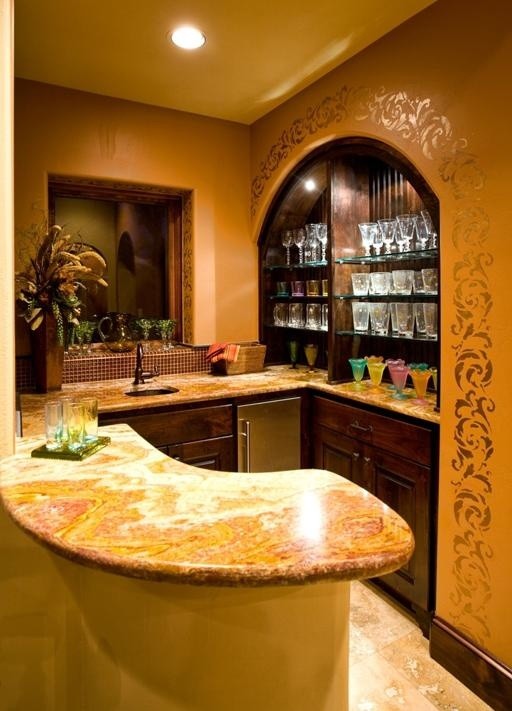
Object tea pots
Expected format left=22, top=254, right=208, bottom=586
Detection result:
left=97, top=311, right=137, bottom=353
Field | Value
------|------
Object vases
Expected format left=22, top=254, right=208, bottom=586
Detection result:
left=75, top=332, right=86, bottom=357
left=85, top=330, right=94, bottom=353
left=141, top=330, right=153, bottom=353
left=159, top=331, right=170, bottom=351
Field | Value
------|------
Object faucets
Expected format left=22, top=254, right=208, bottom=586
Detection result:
left=134, top=342, right=144, bottom=384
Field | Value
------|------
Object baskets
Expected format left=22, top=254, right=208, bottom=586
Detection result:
left=212, top=342, right=267, bottom=376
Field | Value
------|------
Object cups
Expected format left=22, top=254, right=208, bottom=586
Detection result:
left=272, top=270, right=438, bottom=340
left=44, top=394, right=99, bottom=451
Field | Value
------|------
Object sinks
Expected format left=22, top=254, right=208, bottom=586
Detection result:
left=123, top=384, right=178, bottom=397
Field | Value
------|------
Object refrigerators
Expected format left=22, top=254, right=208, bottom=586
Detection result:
left=236, top=396, right=301, bottom=474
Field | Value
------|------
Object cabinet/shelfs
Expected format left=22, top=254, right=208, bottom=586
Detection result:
left=99, top=398, right=234, bottom=472
left=236, top=388, right=310, bottom=473
left=249, top=136, right=441, bottom=412
left=312, top=386, right=439, bottom=641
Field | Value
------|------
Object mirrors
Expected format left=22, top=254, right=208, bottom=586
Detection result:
left=48, top=181, right=182, bottom=345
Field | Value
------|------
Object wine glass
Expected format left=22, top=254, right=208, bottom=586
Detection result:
left=290, top=337, right=438, bottom=407
left=278, top=211, right=438, bottom=265
left=136, top=319, right=177, bottom=352
left=73, top=321, right=99, bottom=357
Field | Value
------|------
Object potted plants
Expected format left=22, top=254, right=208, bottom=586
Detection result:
left=84, top=318, right=97, bottom=338
left=135, top=319, right=156, bottom=338
left=156, top=320, right=173, bottom=336
left=74, top=321, right=89, bottom=339
